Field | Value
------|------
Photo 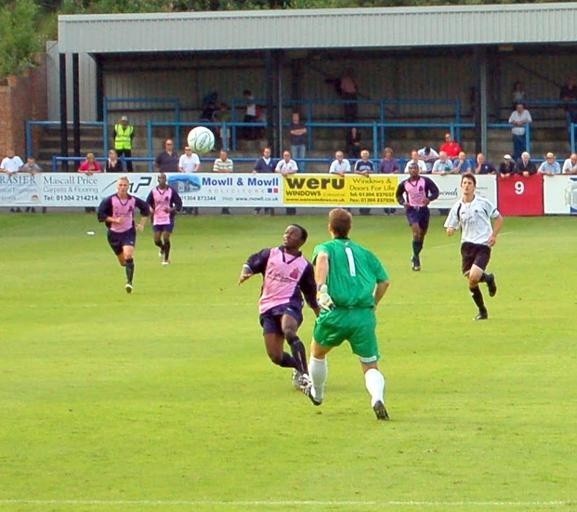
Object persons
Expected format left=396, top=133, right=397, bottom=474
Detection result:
left=238, top=223, right=321, bottom=392
left=202, top=90, right=309, bottom=215
left=329, top=76, right=475, bottom=214
left=146, top=172, right=183, bottom=266
left=96, top=176, right=149, bottom=294
left=76, top=118, right=233, bottom=214
left=474, top=80, right=576, bottom=176
left=443, top=173, right=503, bottom=321
left=299, top=207, right=390, bottom=422
left=1, top=149, right=48, bottom=214
left=396, top=163, right=439, bottom=272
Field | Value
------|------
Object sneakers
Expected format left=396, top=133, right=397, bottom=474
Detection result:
left=488, top=273, right=496, bottom=296
left=126, top=284, right=132, bottom=294
left=371, top=397, right=388, bottom=419
left=473, top=313, right=487, bottom=320
left=298, top=376, right=322, bottom=405
left=162, top=260, right=170, bottom=265
left=412, top=257, right=420, bottom=271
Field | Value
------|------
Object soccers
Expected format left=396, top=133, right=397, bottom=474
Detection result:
left=187, top=126, right=214, bottom=155
